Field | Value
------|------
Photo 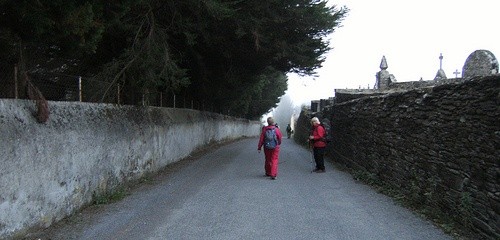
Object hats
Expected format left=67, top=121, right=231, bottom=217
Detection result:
left=312, top=117, right=320, bottom=124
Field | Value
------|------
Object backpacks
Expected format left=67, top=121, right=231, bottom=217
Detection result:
left=320, top=118, right=330, bottom=142
left=265, top=129, right=277, bottom=149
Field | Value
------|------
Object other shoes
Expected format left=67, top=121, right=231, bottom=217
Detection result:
left=272, top=177, right=277, bottom=180
left=267, top=175, right=271, bottom=176
left=313, top=168, right=326, bottom=173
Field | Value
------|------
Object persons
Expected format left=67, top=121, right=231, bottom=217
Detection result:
left=307, top=117, right=327, bottom=173
left=275, top=123, right=291, bottom=139
left=257, top=117, right=284, bottom=180
